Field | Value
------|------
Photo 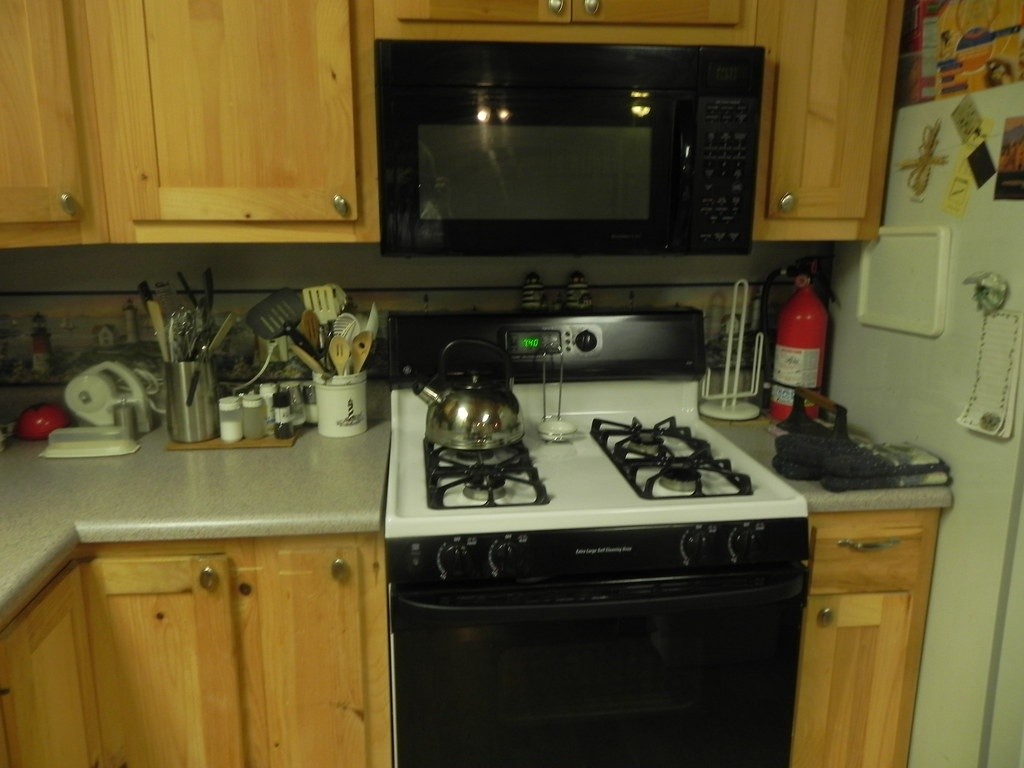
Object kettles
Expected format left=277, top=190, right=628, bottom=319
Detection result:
left=414, top=338, right=524, bottom=449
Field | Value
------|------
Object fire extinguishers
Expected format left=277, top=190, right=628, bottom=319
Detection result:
left=761, top=255, right=843, bottom=426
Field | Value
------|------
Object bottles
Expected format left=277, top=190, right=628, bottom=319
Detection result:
left=302, top=384, right=324, bottom=423
left=281, top=381, right=306, bottom=425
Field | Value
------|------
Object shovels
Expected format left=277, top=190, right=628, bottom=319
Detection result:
left=248, top=286, right=336, bottom=358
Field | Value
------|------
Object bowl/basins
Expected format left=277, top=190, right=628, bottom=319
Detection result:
left=538, top=420, right=577, bottom=442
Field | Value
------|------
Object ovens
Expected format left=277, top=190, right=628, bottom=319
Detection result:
left=388, top=546, right=821, bottom=768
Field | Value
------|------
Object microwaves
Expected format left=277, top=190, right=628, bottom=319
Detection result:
left=375, top=38, right=768, bottom=255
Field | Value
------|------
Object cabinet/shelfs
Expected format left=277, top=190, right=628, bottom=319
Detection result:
left=372, top=0, right=757, bottom=47
left=788, top=508, right=941, bottom=766
left=239, top=535, right=393, bottom=767
left=0, top=1, right=381, bottom=245
left=78, top=538, right=239, bottom=768
left=1, top=561, right=103, bottom=768
left=753, top=0, right=904, bottom=242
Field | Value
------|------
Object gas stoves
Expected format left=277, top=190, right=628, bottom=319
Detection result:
left=383, top=310, right=807, bottom=540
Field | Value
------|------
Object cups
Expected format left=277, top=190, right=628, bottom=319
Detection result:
left=274, top=391, right=293, bottom=437
left=315, top=369, right=370, bottom=439
left=244, top=395, right=265, bottom=441
left=164, top=361, right=218, bottom=442
left=258, top=382, right=279, bottom=423
left=220, top=397, right=242, bottom=442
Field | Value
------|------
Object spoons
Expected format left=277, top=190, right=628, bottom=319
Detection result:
left=329, top=336, right=350, bottom=374
